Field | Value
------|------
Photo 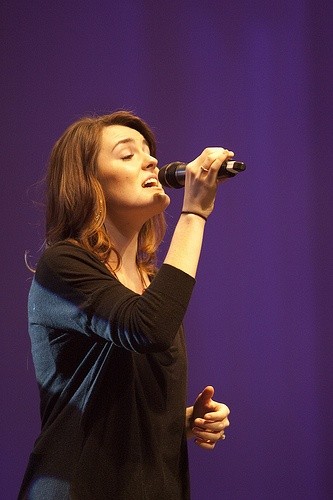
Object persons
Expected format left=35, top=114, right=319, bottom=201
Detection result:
left=16, top=108, right=233, bottom=500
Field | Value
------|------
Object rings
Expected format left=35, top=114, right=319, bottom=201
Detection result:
left=220, top=432, right=226, bottom=440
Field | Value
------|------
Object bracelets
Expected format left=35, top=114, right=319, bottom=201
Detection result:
left=179, top=210, right=207, bottom=223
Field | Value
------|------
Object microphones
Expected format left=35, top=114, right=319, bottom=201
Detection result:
left=158, top=160, right=246, bottom=190
